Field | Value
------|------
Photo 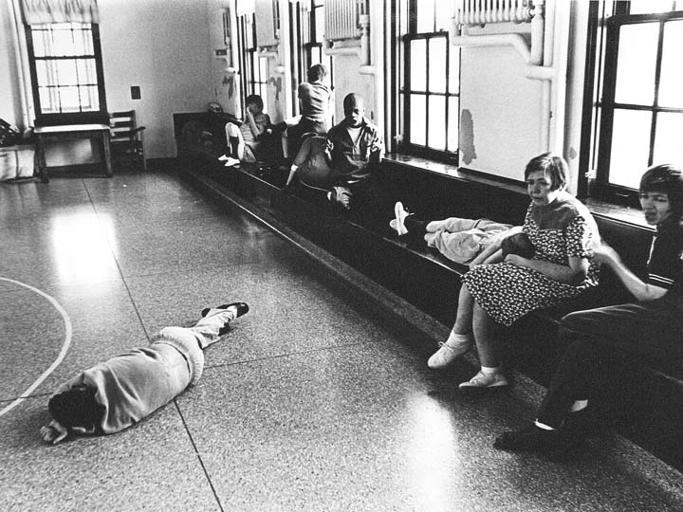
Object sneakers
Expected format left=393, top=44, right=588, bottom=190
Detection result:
left=332, top=185, right=352, bottom=212
left=394, top=201, right=410, bottom=237
left=427, top=335, right=473, bottom=369
left=217, top=154, right=229, bottom=161
left=224, top=156, right=240, bottom=167
left=458, top=371, right=508, bottom=392
left=389, top=219, right=398, bottom=231
left=201, top=301, right=250, bottom=319
left=494, top=423, right=557, bottom=451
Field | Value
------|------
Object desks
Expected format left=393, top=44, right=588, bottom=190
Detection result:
left=33, top=123, right=113, bottom=183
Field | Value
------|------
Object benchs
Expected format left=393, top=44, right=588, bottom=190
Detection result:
left=176, top=133, right=683, bottom=471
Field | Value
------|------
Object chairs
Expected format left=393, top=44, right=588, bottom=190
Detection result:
left=107, top=110, right=147, bottom=173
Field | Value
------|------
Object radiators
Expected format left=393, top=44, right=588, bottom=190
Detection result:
left=324, top=1, right=367, bottom=41
left=460, top=0, right=532, bottom=25
left=211, top=9, right=231, bottom=51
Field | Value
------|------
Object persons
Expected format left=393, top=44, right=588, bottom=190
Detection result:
left=217, top=95, right=270, bottom=168
left=388, top=200, right=536, bottom=269
left=39, top=302, right=249, bottom=444
left=275, top=64, right=333, bottom=167
left=427, top=152, right=602, bottom=391
left=491, top=164, right=683, bottom=454
left=320, top=93, right=382, bottom=215
